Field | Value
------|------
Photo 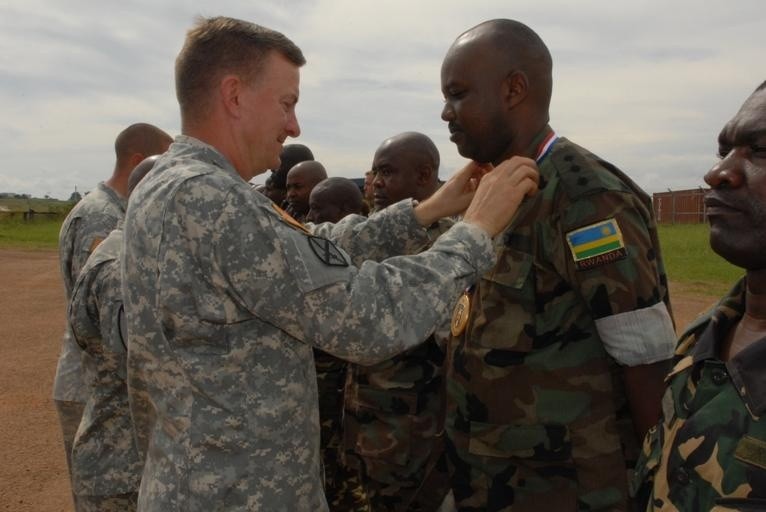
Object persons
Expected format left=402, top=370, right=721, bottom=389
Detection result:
left=622, top=79, right=766, bottom=512
left=51, top=124, right=173, bottom=512
left=70, top=156, right=162, bottom=512
left=249, top=131, right=452, bottom=511
left=120, top=16, right=539, bottom=512
left=442, top=19, right=677, bottom=512
